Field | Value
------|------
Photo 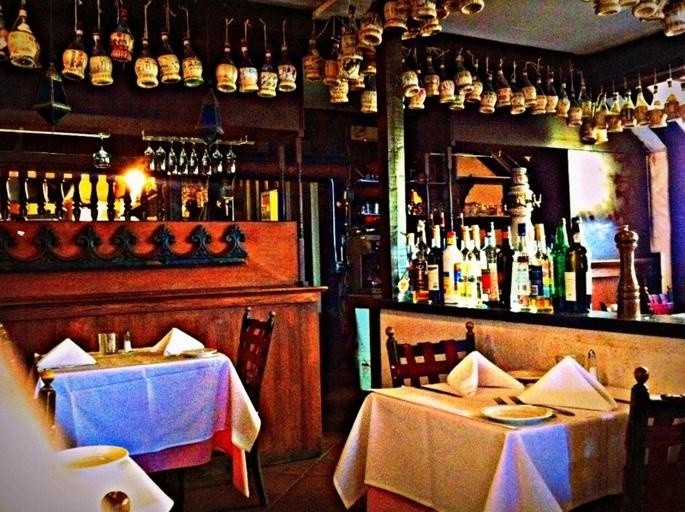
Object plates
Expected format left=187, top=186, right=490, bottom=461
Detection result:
left=55, top=447, right=128, bottom=474
left=183, top=347, right=222, bottom=359
left=481, top=406, right=556, bottom=425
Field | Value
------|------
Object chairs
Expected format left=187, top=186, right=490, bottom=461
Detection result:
left=176, top=307, right=276, bottom=505
left=622, top=367, right=685, bottom=512
left=385, top=321, right=475, bottom=387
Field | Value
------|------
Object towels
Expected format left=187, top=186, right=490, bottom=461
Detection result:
left=153, top=327, right=204, bottom=357
left=447, top=350, right=525, bottom=398
left=36, top=338, right=96, bottom=369
left=516, top=355, right=619, bottom=411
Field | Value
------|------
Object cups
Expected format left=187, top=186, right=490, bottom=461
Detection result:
left=556, top=352, right=585, bottom=375
left=96, top=331, right=117, bottom=356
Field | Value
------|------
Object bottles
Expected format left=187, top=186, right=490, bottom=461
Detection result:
left=615, top=223, right=642, bottom=320
left=406, top=217, right=592, bottom=317
left=123, top=331, right=134, bottom=356
left=0, top=0, right=685, bottom=146
left=587, top=350, right=599, bottom=387
left=1, top=170, right=173, bottom=221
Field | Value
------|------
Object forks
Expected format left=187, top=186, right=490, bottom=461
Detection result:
left=493, top=394, right=576, bottom=419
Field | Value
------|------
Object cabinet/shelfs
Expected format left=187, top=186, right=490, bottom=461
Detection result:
left=350, top=151, right=511, bottom=295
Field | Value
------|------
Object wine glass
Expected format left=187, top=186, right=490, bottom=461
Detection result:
left=143, top=136, right=243, bottom=176
left=88, top=130, right=114, bottom=171
